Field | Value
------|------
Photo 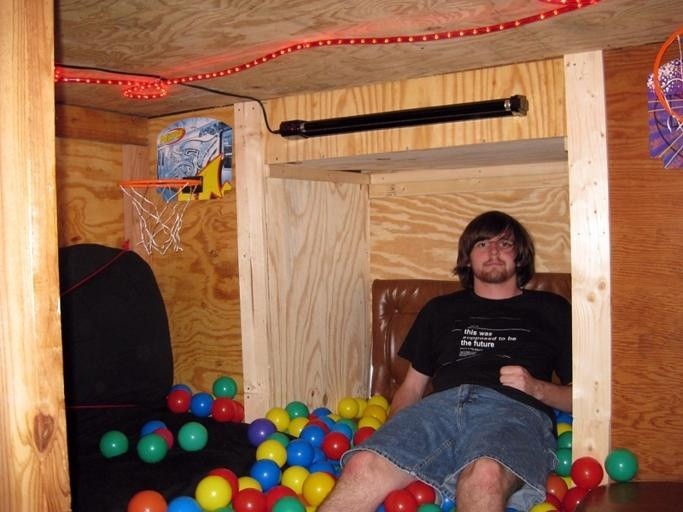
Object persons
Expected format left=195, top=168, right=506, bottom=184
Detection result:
left=315, top=209, right=572, bottom=511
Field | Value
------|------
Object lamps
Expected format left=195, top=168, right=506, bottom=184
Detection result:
left=278, top=95, right=530, bottom=143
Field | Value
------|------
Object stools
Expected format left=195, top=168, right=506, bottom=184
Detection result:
left=576, top=479, right=682, bottom=511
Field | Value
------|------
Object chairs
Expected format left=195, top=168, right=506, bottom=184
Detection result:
left=59, top=241, right=295, bottom=512
left=372, top=272, right=571, bottom=412
